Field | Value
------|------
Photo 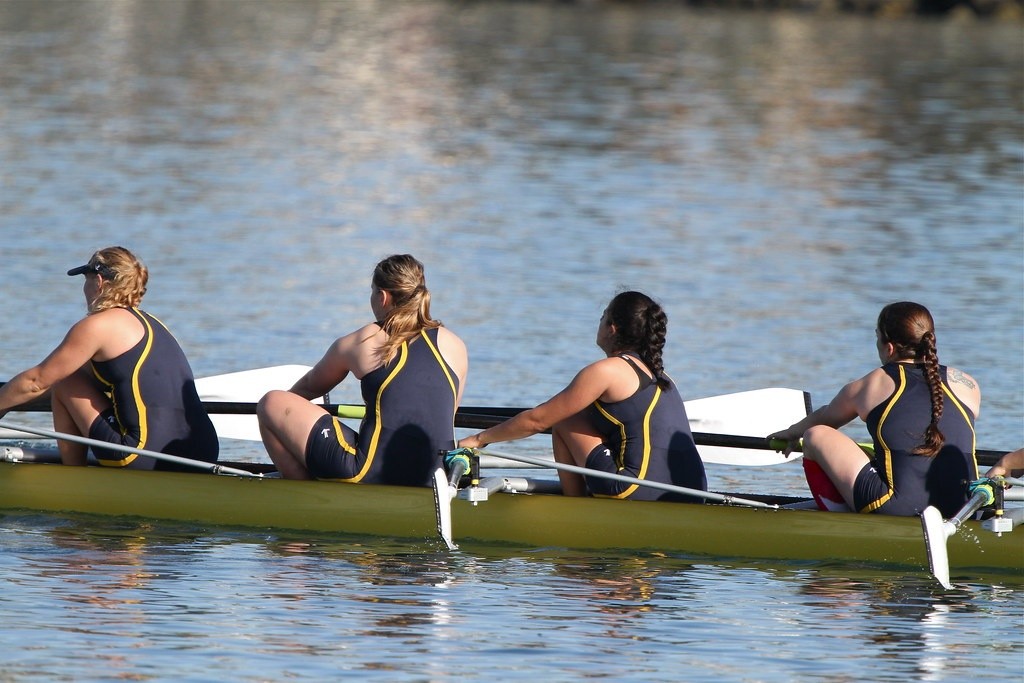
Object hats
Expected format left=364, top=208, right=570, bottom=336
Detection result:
left=67, top=254, right=146, bottom=296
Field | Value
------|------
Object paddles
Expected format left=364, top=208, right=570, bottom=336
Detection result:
left=2, top=385, right=816, bottom=468
left=430, top=446, right=473, bottom=550
left=917, top=473, right=1009, bottom=589
left=313, top=402, right=806, bottom=455
left=0, top=358, right=331, bottom=443
left=857, top=440, right=1013, bottom=467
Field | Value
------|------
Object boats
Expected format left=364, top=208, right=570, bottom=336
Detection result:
left=1, top=417, right=1024, bottom=575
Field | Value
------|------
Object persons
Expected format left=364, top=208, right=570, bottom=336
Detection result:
left=0, top=246, right=219, bottom=473
left=765, top=301, right=981, bottom=520
left=457, top=291, right=708, bottom=505
left=257, top=254, right=467, bottom=488
left=984, top=448, right=1024, bottom=490
